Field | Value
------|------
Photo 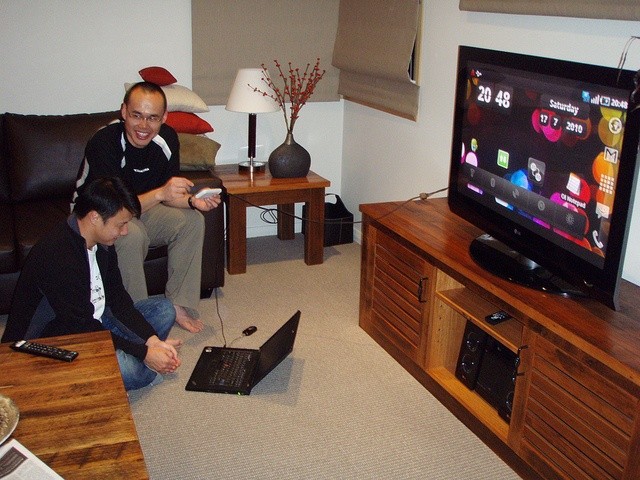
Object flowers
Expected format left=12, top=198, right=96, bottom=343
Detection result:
left=248, top=57, right=327, bottom=135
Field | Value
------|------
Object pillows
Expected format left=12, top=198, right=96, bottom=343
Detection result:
left=124, top=84, right=211, bottom=111
left=175, top=133, right=222, bottom=172
left=164, top=111, right=214, bottom=133
left=140, top=67, right=180, bottom=85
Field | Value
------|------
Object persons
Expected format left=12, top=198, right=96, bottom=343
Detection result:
left=1, top=175, right=183, bottom=391
left=67, top=81, right=222, bottom=334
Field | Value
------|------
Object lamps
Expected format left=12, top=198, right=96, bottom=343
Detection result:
left=225, top=70, right=284, bottom=175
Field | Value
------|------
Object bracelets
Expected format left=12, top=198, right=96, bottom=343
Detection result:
left=188, top=193, right=196, bottom=210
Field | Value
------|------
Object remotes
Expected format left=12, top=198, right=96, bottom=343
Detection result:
left=9, top=339, right=78, bottom=362
left=487, top=312, right=512, bottom=324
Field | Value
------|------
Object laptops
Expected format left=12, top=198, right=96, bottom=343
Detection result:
left=186, top=311, right=300, bottom=395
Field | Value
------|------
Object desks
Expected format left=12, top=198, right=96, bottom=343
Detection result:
left=0, top=330, right=143, bottom=474
left=210, top=162, right=335, bottom=274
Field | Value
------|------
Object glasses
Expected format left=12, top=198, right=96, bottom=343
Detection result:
left=127, top=105, right=165, bottom=122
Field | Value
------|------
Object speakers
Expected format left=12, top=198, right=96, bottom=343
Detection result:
left=454, top=320, right=519, bottom=425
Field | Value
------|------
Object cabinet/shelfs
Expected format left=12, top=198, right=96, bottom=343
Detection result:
left=357, top=196, right=638, bottom=462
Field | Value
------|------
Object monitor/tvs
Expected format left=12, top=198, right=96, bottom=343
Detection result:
left=448, top=44, right=639, bottom=312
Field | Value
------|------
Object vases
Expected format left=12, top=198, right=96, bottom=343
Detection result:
left=266, top=134, right=314, bottom=178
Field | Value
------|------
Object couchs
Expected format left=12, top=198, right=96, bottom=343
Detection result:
left=4, top=111, right=222, bottom=314
